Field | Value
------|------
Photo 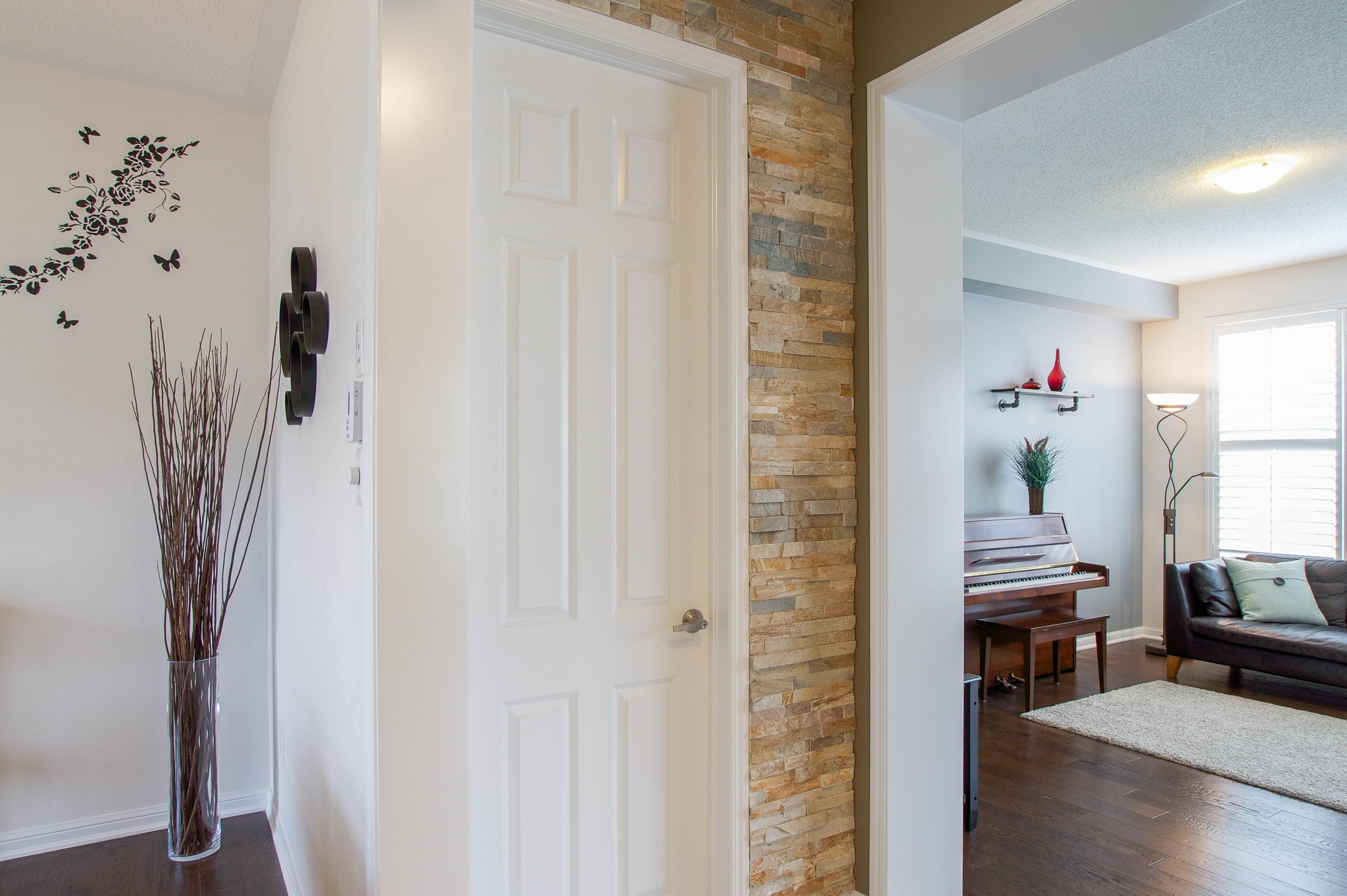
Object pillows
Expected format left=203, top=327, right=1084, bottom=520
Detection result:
left=1221, top=554, right=1332, bottom=630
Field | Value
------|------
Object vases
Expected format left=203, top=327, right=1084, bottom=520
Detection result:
left=1047, top=346, right=1069, bottom=392
left=165, top=653, right=221, bottom=862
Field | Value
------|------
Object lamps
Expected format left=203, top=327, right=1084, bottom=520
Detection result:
left=1144, top=389, right=1223, bottom=658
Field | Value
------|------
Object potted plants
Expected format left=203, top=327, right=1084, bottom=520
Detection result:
left=998, top=427, right=1075, bottom=515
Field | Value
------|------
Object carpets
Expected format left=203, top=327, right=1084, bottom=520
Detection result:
left=1016, top=679, right=1347, bottom=815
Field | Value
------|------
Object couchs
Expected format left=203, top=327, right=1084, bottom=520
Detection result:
left=1160, top=551, right=1347, bottom=682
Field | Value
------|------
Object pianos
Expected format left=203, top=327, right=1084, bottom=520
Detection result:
left=964, top=512, right=1110, bottom=692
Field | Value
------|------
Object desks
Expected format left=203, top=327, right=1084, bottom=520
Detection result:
left=963, top=672, right=983, bottom=834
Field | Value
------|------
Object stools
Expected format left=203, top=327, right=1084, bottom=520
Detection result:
left=975, top=602, right=1112, bottom=714
